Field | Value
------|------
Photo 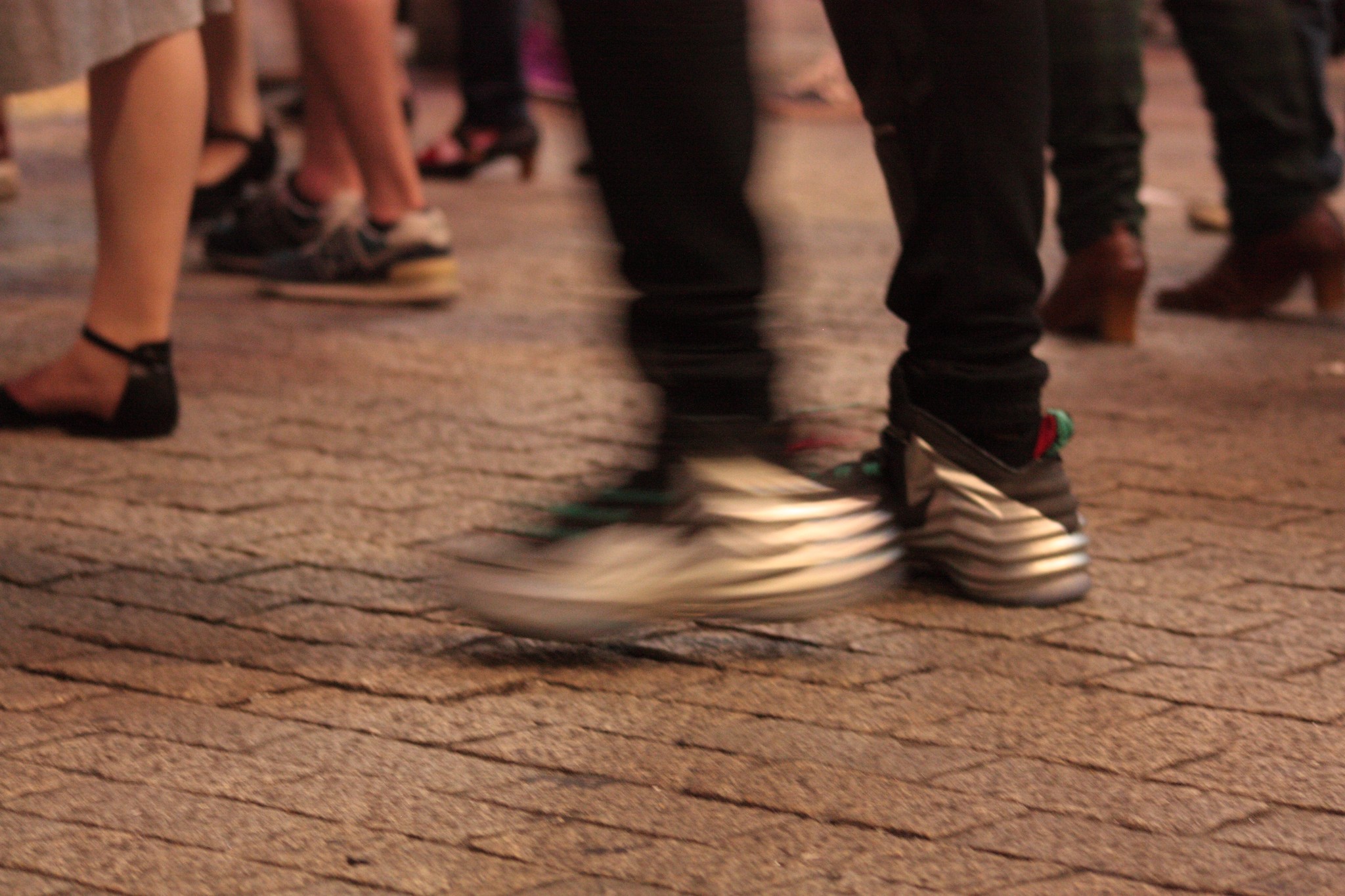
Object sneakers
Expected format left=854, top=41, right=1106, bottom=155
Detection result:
left=254, top=204, right=465, bottom=303
left=419, top=427, right=913, bottom=642
left=202, top=169, right=365, bottom=270
left=775, top=391, right=1096, bottom=608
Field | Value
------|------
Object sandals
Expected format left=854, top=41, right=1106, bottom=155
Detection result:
left=0, top=326, right=183, bottom=441
left=186, top=123, right=281, bottom=221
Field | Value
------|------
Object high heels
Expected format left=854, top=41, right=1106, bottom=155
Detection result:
left=1041, top=228, right=1148, bottom=343
left=1155, top=199, right=1344, bottom=319
left=414, top=119, right=540, bottom=184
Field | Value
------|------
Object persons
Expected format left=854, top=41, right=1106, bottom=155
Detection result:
left=412, top=2, right=542, bottom=185
left=417, top=1, right=1098, bottom=635
left=199, top=2, right=464, bottom=309
left=1035, top=0, right=1345, bottom=343
left=1, top=1, right=214, bottom=442
left=189, top=3, right=280, bottom=224
